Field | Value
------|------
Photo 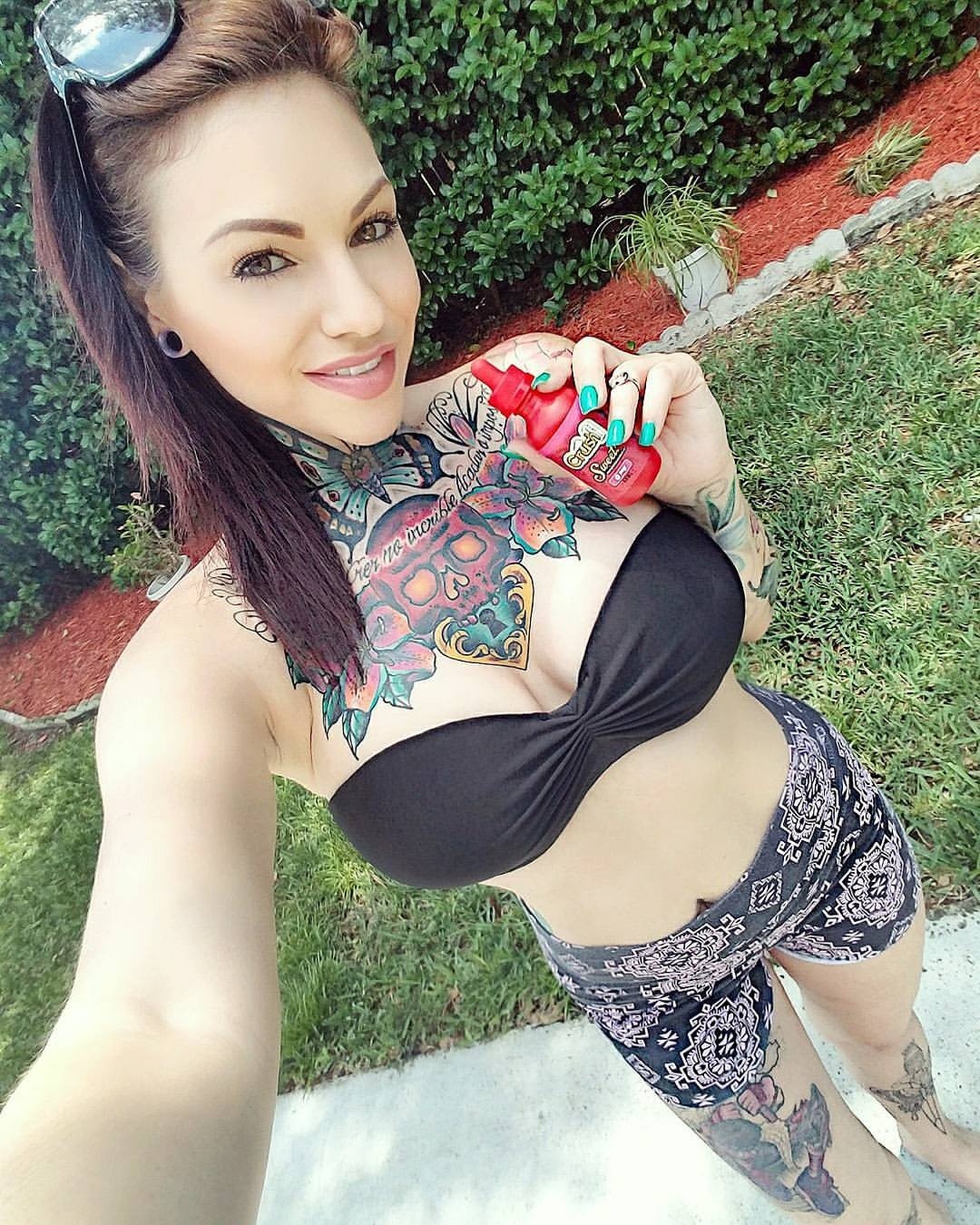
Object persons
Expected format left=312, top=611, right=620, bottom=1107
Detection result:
left=0, top=0, right=980, bottom=1225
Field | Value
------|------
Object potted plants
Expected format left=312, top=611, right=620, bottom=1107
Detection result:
left=101, top=492, right=192, bottom=606
left=589, top=178, right=745, bottom=317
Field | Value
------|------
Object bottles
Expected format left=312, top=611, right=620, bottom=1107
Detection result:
left=470, top=358, right=661, bottom=508
